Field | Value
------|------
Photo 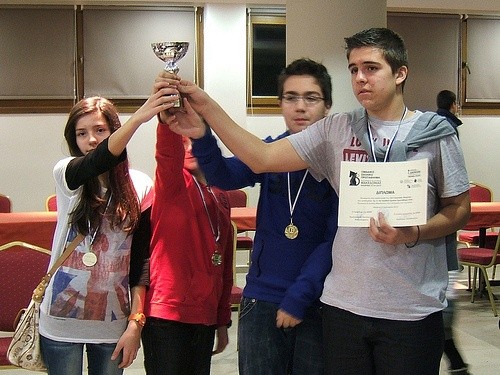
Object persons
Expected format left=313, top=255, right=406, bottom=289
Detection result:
left=435, top=88, right=466, bottom=273
left=160, top=57, right=339, bottom=375
left=178, top=27, right=474, bottom=375
left=37, top=95, right=147, bottom=375
left=140, top=64, right=238, bottom=375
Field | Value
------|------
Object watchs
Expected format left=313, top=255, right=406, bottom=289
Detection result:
left=128, top=311, right=146, bottom=327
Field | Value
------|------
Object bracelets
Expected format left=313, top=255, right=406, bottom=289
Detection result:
left=405, top=223, right=420, bottom=249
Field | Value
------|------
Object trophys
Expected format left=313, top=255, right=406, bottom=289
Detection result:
left=152, top=42, right=190, bottom=109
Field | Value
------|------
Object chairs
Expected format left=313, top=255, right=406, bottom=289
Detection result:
left=454, top=181, right=500, bottom=317
left=0, top=187, right=254, bottom=370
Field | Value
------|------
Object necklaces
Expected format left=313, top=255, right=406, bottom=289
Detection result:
left=364, top=106, right=408, bottom=162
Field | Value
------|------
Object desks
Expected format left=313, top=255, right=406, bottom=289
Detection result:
left=455, top=202, right=500, bottom=300
left=0, top=203, right=257, bottom=300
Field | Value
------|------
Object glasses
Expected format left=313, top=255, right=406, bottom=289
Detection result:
left=281, top=94, right=324, bottom=105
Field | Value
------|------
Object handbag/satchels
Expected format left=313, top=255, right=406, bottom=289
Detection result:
left=6, top=294, right=48, bottom=372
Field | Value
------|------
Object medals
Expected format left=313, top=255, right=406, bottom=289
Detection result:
left=82, top=253, right=98, bottom=267
left=284, top=224, right=299, bottom=240
left=211, top=250, right=223, bottom=267
